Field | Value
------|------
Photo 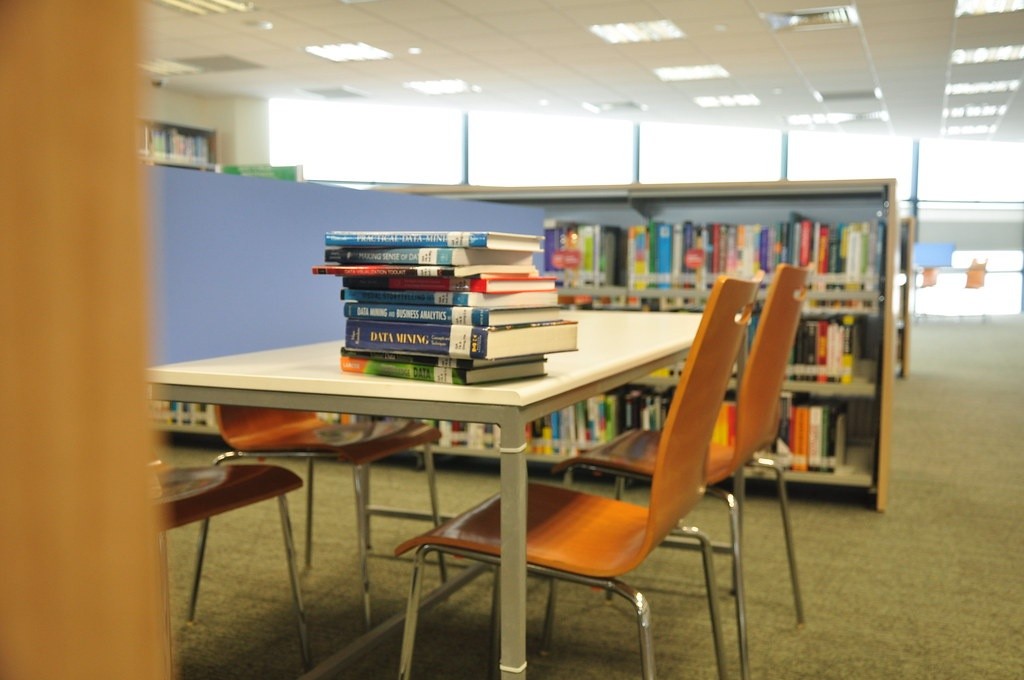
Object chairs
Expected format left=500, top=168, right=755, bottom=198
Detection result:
left=157, top=463, right=311, bottom=673
left=188, top=402, right=447, bottom=630
left=540, top=262, right=818, bottom=680
left=393, top=269, right=768, bottom=680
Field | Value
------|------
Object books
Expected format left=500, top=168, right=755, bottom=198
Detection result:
left=310, top=228, right=576, bottom=385
left=155, top=391, right=850, bottom=475
left=139, top=124, right=209, bottom=165
left=578, top=219, right=881, bottom=311
left=646, top=318, right=866, bottom=384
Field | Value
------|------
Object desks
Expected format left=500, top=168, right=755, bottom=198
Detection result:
left=144, top=309, right=752, bottom=680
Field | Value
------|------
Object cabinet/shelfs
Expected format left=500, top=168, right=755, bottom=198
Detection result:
left=158, top=172, right=904, bottom=516
